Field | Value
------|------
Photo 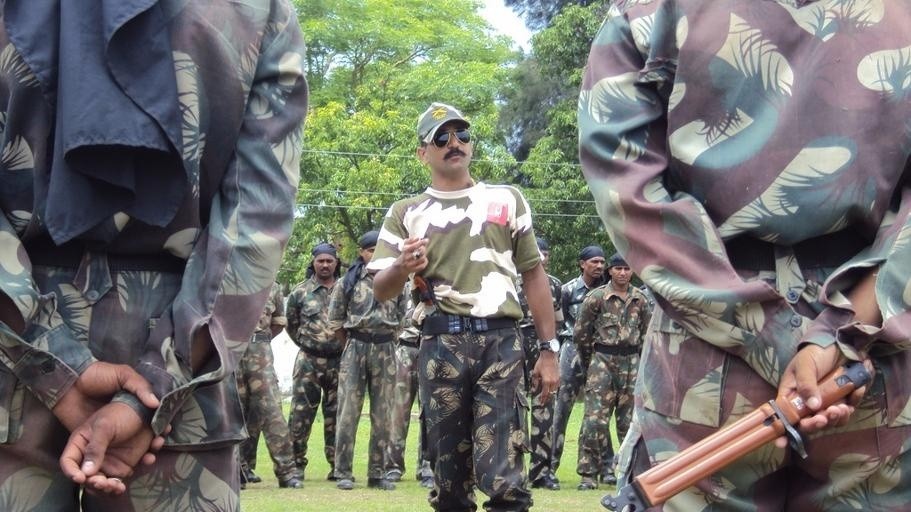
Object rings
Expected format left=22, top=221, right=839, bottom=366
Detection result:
left=412, top=249, right=420, bottom=260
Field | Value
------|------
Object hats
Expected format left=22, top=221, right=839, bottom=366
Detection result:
left=417, top=102, right=471, bottom=145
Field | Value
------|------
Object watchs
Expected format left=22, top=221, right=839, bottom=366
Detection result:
left=538, top=338, right=560, bottom=355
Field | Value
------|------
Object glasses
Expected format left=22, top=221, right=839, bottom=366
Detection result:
left=433, top=129, right=471, bottom=149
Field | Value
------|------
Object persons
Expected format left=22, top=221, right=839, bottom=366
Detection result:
left=576, top=253, right=651, bottom=492
left=284, top=242, right=357, bottom=482
left=549, top=246, right=616, bottom=484
left=366, top=104, right=561, bottom=505
left=330, top=232, right=405, bottom=492
left=514, top=238, right=551, bottom=489
left=231, top=281, right=304, bottom=490
left=577, top=1, right=911, bottom=512
left=385, top=265, right=437, bottom=483
left=1, top=1, right=307, bottom=511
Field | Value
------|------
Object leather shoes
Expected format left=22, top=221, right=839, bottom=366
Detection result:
left=280, top=479, right=303, bottom=488
left=579, top=482, right=592, bottom=490
left=389, top=472, right=401, bottom=482
left=367, top=478, right=395, bottom=490
left=328, top=472, right=355, bottom=482
left=295, top=468, right=304, bottom=481
left=550, top=474, right=559, bottom=483
left=600, top=474, right=617, bottom=484
left=543, top=480, right=560, bottom=490
left=338, top=481, right=354, bottom=490
left=248, top=473, right=262, bottom=483
left=422, top=477, right=434, bottom=488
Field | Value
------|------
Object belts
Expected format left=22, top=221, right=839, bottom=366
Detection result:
left=422, top=315, right=516, bottom=336
left=300, top=343, right=342, bottom=359
left=522, top=327, right=536, bottom=337
left=399, top=338, right=420, bottom=347
left=566, top=336, right=572, bottom=341
left=22, top=240, right=187, bottom=272
left=727, top=235, right=869, bottom=270
left=594, top=343, right=639, bottom=355
left=250, top=334, right=272, bottom=343
left=349, top=330, right=394, bottom=343
